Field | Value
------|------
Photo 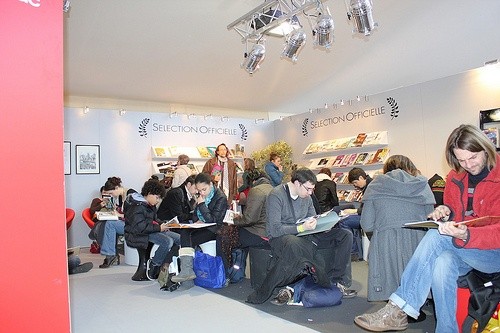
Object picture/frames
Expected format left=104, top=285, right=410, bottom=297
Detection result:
left=64, top=140, right=72, bottom=176
left=74, top=144, right=100, bottom=174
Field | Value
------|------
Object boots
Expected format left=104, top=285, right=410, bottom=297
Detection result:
left=170, top=247, right=197, bottom=283
left=68, top=254, right=94, bottom=275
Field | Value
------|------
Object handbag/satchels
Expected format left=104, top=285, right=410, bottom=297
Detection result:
left=192, top=251, right=224, bottom=288
left=90, top=241, right=100, bottom=253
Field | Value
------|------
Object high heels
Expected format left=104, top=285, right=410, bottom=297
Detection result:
left=100, top=254, right=120, bottom=268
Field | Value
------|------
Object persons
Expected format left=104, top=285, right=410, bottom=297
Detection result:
left=236, top=151, right=285, bottom=206
left=354, top=124, right=500, bottom=333
left=171, top=175, right=229, bottom=292
left=314, top=168, right=355, bottom=215
left=338, top=168, right=373, bottom=261
left=360, top=155, right=436, bottom=324
left=221, top=169, right=274, bottom=287
left=123, top=180, right=181, bottom=280
left=157, top=155, right=199, bottom=220
left=266, top=169, right=358, bottom=306
left=89, top=177, right=138, bottom=268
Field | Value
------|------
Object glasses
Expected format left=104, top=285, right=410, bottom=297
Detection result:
left=298, top=181, right=316, bottom=192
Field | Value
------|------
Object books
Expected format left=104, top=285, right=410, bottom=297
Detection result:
left=295, top=210, right=349, bottom=237
left=401, top=216, right=500, bottom=229
left=233, top=162, right=244, bottom=173
left=305, top=132, right=390, bottom=169
left=164, top=216, right=217, bottom=229
left=152, top=144, right=245, bottom=159
left=222, top=210, right=241, bottom=225
left=156, top=161, right=225, bottom=176
left=331, top=172, right=363, bottom=202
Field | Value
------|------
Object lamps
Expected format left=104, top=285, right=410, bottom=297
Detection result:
left=240, top=33, right=266, bottom=77
left=301, top=0, right=334, bottom=48
left=280, top=16, right=307, bottom=63
left=345, top=0, right=379, bottom=37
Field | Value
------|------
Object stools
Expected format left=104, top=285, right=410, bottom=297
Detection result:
left=126, top=240, right=153, bottom=282
left=249, top=247, right=274, bottom=289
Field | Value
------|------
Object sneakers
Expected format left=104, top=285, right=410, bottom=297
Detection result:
left=353, top=302, right=409, bottom=331
left=146, top=259, right=158, bottom=281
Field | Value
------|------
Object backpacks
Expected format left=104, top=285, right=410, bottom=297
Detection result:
left=227, top=247, right=246, bottom=284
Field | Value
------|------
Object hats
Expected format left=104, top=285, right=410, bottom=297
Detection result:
left=238, top=170, right=265, bottom=192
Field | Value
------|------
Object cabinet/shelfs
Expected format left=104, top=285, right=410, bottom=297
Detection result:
left=308, top=144, right=391, bottom=201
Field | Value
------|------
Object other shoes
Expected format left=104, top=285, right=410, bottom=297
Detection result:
left=351, top=253, right=359, bottom=262
left=335, top=282, right=357, bottom=299
left=271, top=288, right=295, bottom=306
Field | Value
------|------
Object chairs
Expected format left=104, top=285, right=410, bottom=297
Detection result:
left=66, top=208, right=76, bottom=230
left=83, top=207, right=96, bottom=228
